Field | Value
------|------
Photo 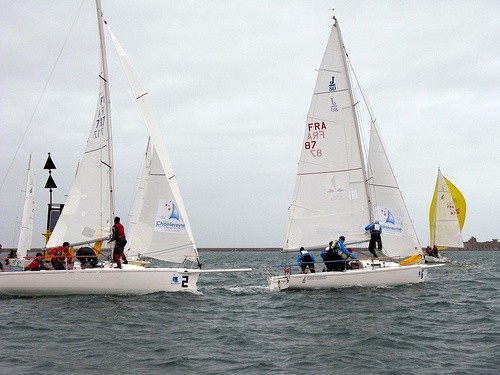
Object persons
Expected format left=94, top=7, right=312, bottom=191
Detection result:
left=364, top=220, right=383, bottom=260
left=425, top=245, right=439, bottom=258
left=106, top=216, right=128, bottom=269
left=320, top=236, right=357, bottom=272
left=296, top=246, right=316, bottom=274
left=0, top=241, right=98, bottom=272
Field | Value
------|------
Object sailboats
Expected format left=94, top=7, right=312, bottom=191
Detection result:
left=424, top=166, right=467, bottom=265
left=267, top=8, right=446, bottom=291
left=0, top=1, right=254, bottom=296
